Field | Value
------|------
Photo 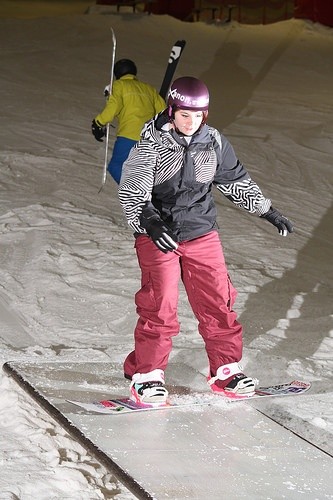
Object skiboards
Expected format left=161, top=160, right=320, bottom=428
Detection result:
left=99, top=26, right=186, bottom=192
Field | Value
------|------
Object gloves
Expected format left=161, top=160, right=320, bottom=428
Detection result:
left=144, top=216, right=178, bottom=254
left=91, top=118, right=107, bottom=143
left=258, top=204, right=294, bottom=236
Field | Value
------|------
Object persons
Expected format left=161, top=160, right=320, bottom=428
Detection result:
left=91, top=59, right=169, bottom=186
left=118, top=77, right=295, bottom=406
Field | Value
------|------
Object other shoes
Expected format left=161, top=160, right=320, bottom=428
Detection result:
left=128, top=368, right=168, bottom=403
left=205, top=362, right=255, bottom=395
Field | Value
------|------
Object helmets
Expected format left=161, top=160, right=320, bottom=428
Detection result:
left=113, top=58, right=137, bottom=80
left=166, top=76, right=209, bottom=111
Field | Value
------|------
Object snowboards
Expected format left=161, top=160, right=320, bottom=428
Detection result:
left=64, top=361, right=312, bottom=415
